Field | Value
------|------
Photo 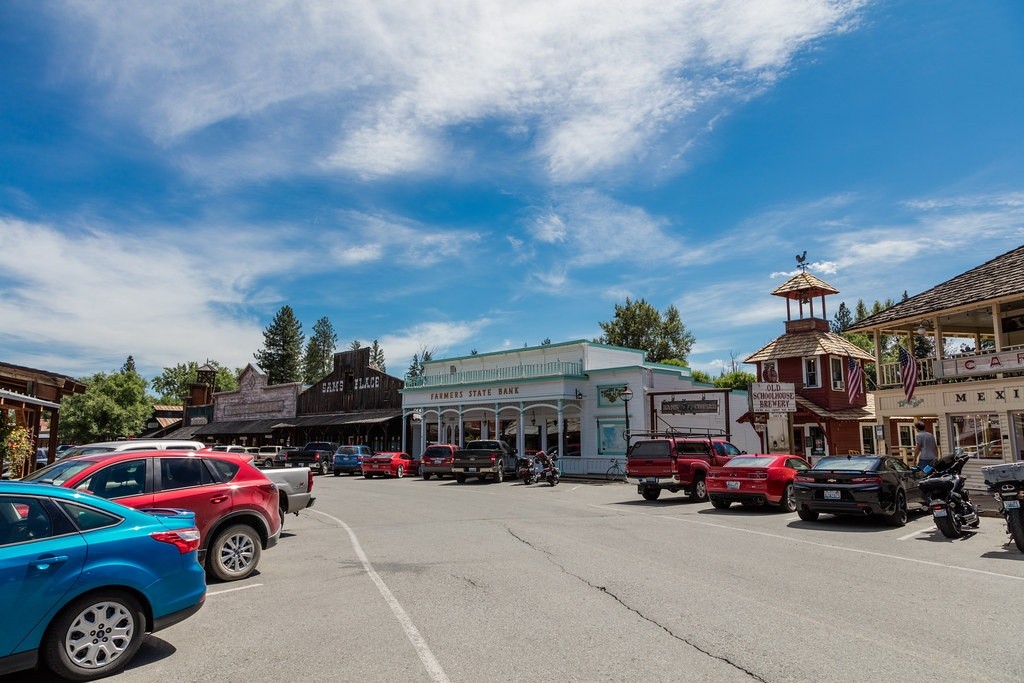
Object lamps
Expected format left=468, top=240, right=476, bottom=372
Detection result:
left=917, top=318, right=936, bottom=335
left=575, top=388, right=582, bottom=400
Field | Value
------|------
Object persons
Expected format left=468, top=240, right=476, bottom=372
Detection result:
left=913, top=421, right=939, bottom=470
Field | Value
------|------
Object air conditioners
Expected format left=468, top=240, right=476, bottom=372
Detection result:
left=834, top=381, right=845, bottom=390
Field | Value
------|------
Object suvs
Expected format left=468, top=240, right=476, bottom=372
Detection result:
left=623, top=427, right=747, bottom=502
left=421, top=444, right=460, bottom=480
left=19, top=446, right=283, bottom=581
left=332, top=445, right=373, bottom=476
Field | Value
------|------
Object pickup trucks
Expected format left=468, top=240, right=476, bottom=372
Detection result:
left=284, top=441, right=341, bottom=475
left=450, top=439, right=524, bottom=484
left=46, top=439, right=317, bottom=536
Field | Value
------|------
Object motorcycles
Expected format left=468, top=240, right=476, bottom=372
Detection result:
left=984, top=478, right=1024, bottom=553
left=518, top=454, right=539, bottom=484
left=536, top=450, right=560, bottom=486
left=919, top=448, right=982, bottom=538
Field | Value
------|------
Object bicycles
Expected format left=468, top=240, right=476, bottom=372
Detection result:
left=606, top=458, right=629, bottom=482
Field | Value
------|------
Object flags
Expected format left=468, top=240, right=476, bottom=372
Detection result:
left=898, top=344, right=919, bottom=404
left=847, top=353, right=862, bottom=407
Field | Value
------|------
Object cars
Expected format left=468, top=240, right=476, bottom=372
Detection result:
left=31, top=444, right=73, bottom=466
left=362, top=452, right=422, bottom=478
left=212, top=441, right=303, bottom=472
left=953, top=440, right=1002, bottom=459
left=792, top=455, right=933, bottom=526
left=706, top=453, right=814, bottom=512
left=0, top=478, right=208, bottom=683
